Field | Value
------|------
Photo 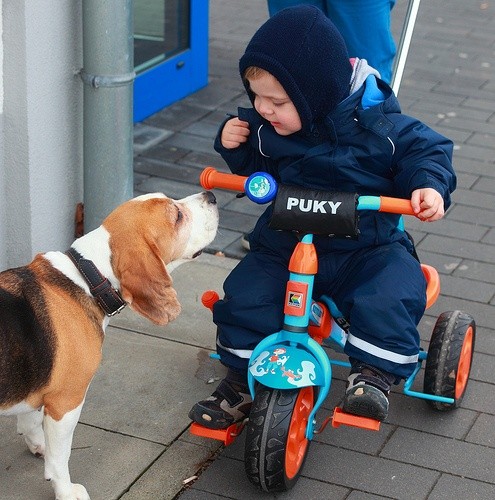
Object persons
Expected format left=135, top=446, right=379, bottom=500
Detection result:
left=266, top=0, right=396, bottom=85
left=187, top=6, right=457, bottom=430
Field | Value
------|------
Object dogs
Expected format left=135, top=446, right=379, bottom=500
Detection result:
left=0, top=190, right=219, bottom=500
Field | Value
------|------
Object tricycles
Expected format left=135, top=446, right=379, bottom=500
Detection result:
left=190, top=167, right=476, bottom=493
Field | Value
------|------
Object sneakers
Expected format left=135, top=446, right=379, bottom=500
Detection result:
left=344, top=366, right=390, bottom=421
left=188, top=378, right=253, bottom=429
